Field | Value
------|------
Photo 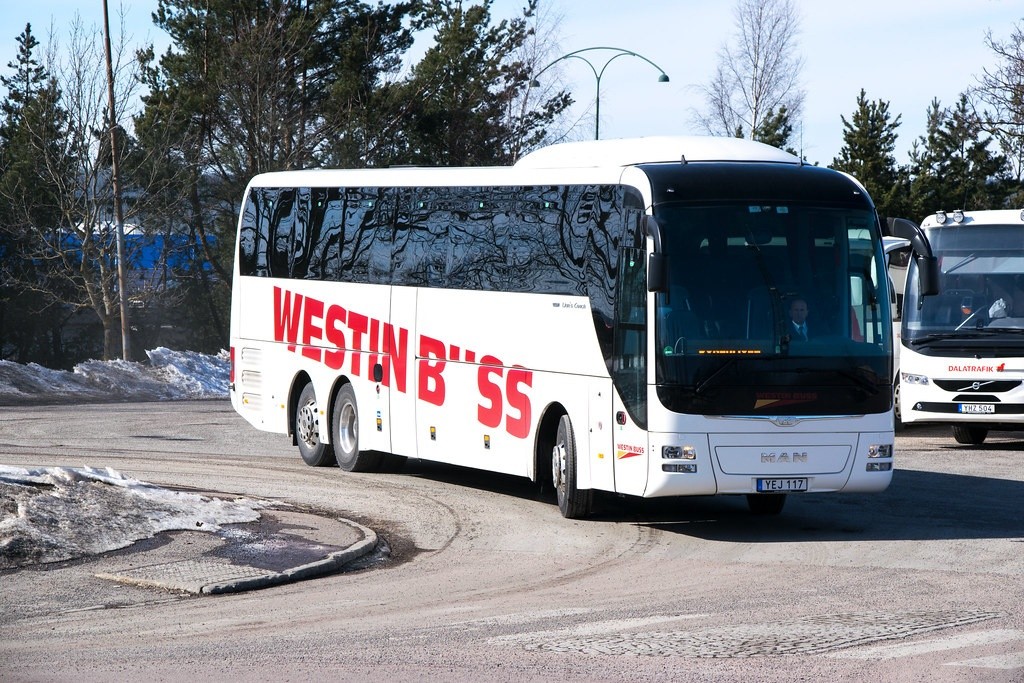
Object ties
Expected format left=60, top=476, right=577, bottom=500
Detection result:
left=798, top=326, right=806, bottom=342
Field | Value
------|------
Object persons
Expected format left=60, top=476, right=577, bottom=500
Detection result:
left=781, top=300, right=819, bottom=344
left=989, top=274, right=1024, bottom=318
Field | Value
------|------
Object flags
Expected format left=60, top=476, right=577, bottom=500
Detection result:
left=852, top=307, right=864, bottom=341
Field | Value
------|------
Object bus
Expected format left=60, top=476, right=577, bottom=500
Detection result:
left=880, top=208, right=1024, bottom=445
left=227, top=135, right=941, bottom=518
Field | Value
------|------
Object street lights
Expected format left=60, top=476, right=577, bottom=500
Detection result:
left=529, top=45, right=671, bottom=141
